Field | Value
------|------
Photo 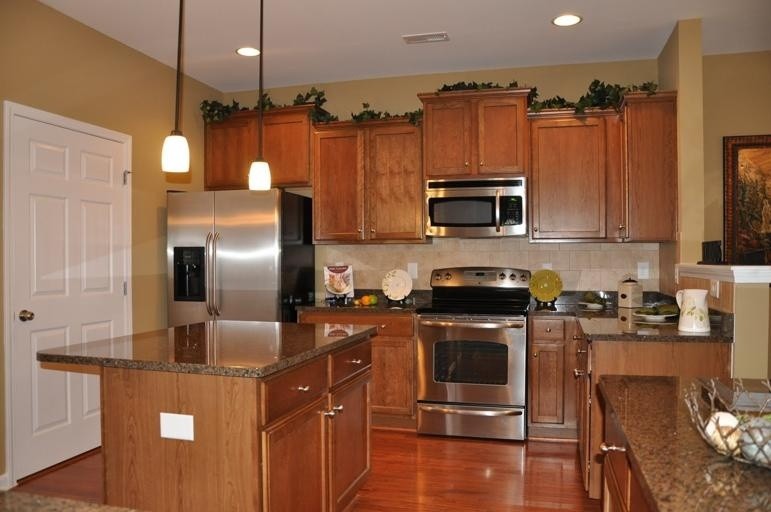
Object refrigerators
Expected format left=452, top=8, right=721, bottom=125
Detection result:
left=165, top=187, right=315, bottom=321
left=169, top=321, right=282, bottom=364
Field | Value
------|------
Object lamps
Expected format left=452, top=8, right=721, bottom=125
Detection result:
left=160, top=0, right=190, bottom=173
left=248, top=0, right=271, bottom=192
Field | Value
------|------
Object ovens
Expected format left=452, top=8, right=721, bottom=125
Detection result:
left=414, top=316, right=527, bottom=441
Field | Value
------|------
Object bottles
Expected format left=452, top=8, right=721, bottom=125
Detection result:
left=617, top=308, right=642, bottom=333
left=618, top=278, right=644, bottom=308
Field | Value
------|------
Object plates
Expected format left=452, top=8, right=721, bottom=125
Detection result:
left=633, top=309, right=678, bottom=320
left=528, top=270, right=564, bottom=303
left=381, top=270, right=413, bottom=300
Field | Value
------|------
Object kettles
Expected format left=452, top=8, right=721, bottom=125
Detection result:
left=676, top=289, right=711, bottom=332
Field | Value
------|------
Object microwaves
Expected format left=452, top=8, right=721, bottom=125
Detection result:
left=427, top=177, right=529, bottom=237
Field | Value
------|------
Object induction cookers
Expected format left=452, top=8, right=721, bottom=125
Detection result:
left=416, top=299, right=529, bottom=314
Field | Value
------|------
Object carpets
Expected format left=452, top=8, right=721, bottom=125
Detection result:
left=0, top=489, right=137, bottom=512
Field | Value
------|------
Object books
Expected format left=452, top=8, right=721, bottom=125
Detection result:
left=714, top=378, right=771, bottom=413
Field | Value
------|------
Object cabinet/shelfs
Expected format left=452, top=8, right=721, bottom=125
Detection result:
left=203, top=102, right=330, bottom=190
left=415, top=85, right=531, bottom=178
left=309, top=115, right=432, bottom=244
left=526, top=90, right=677, bottom=245
left=600, top=392, right=657, bottom=512
left=527, top=315, right=731, bottom=501
left=296, top=309, right=417, bottom=432
left=101, top=336, right=373, bottom=512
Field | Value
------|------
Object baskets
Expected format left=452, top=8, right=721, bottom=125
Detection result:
left=682, top=377, right=770, bottom=468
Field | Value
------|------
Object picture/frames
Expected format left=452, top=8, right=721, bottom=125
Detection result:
left=723, top=134, right=771, bottom=265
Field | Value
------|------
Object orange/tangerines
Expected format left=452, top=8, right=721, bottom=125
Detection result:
left=370, top=294, right=378, bottom=305
